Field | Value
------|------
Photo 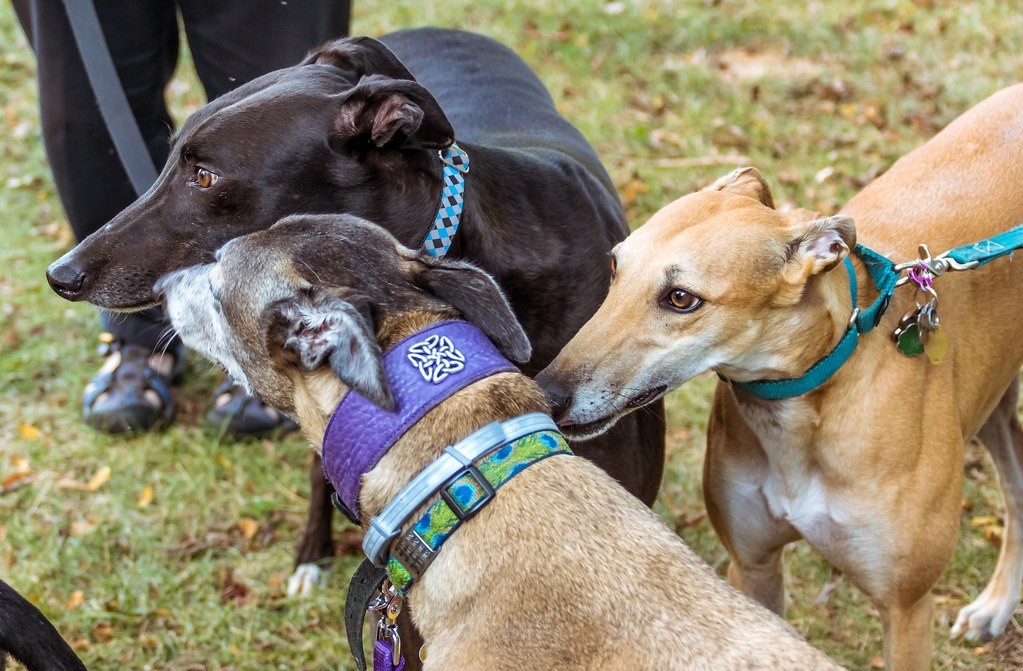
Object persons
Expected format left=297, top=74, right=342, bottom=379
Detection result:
left=12, top=1, right=353, bottom=434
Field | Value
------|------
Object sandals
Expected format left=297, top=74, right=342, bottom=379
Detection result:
left=204, top=376, right=299, bottom=443
left=81, top=335, right=183, bottom=442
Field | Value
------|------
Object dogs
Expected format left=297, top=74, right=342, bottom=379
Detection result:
left=153, top=212, right=848, bottom=671
left=45, top=27, right=667, bottom=671
left=535, top=82, right=1023, bottom=671
